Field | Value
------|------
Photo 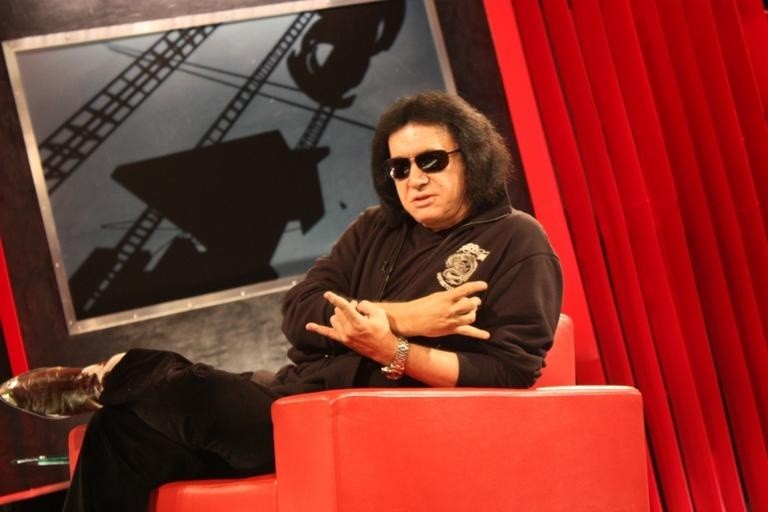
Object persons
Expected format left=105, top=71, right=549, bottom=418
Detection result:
left=0, top=89, right=566, bottom=508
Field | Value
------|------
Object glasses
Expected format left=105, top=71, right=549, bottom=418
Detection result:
left=389, top=150, right=460, bottom=179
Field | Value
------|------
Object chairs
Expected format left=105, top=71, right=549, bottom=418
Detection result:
left=68, top=312, right=650, bottom=512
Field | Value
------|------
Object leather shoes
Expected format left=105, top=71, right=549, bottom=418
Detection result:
left=0, top=367, right=102, bottom=420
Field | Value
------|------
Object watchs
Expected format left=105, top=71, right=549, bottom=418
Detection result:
left=379, top=337, right=412, bottom=383
